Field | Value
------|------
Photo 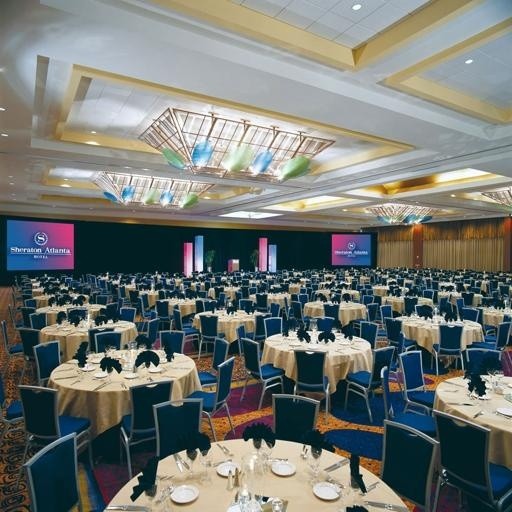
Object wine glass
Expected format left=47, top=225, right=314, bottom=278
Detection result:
left=236, top=484, right=284, bottom=512
left=250, top=441, right=273, bottom=473
left=308, top=452, right=322, bottom=486
left=199, top=449, right=215, bottom=483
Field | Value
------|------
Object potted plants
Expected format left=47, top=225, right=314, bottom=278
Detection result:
left=248, top=248, right=259, bottom=272
left=204, top=249, right=216, bottom=272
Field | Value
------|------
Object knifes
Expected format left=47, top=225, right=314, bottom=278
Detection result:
left=106, top=504, right=152, bottom=511
left=215, top=442, right=235, bottom=456
left=442, top=370, right=482, bottom=422
left=361, top=480, right=382, bottom=495
left=362, top=501, right=408, bottom=511
left=174, top=453, right=189, bottom=471
left=325, top=457, right=351, bottom=472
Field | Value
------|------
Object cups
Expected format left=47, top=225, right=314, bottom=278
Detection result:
left=487, top=368, right=506, bottom=388
left=86, top=340, right=165, bottom=366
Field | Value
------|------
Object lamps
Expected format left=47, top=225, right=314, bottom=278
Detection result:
left=364, top=200, right=443, bottom=226
left=87, top=169, right=215, bottom=209
left=137, top=107, right=336, bottom=183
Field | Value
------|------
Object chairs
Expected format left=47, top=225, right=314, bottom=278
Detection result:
left=1, top=266, right=511, bottom=512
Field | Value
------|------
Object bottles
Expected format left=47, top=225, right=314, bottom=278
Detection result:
left=226, top=467, right=239, bottom=491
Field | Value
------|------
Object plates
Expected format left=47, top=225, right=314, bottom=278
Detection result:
left=396, top=312, right=476, bottom=328
left=470, top=378, right=512, bottom=418
left=217, top=461, right=242, bottom=477
left=81, top=354, right=168, bottom=378
left=272, top=460, right=297, bottom=476
left=313, top=482, right=341, bottom=500
left=170, top=484, right=199, bottom=503
left=285, top=330, right=354, bottom=351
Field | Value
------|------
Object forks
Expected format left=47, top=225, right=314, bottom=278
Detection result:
left=300, top=444, right=308, bottom=461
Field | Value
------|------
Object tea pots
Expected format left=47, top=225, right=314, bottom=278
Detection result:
left=307, top=319, right=319, bottom=331
